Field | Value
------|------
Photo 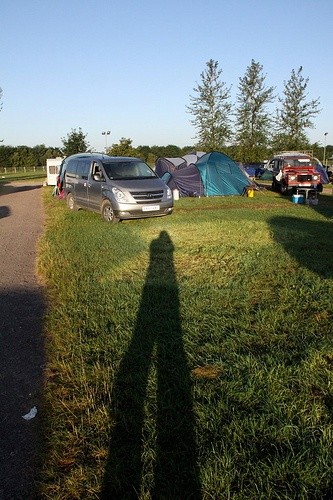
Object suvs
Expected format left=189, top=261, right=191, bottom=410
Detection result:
left=272, top=150, right=323, bottom=195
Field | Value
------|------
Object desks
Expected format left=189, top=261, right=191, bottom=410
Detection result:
left=296, top=187, right=318, bottom=205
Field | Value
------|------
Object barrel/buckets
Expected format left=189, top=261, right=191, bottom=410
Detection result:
left=292, top=194, right=303, bottom=203
left=173, top=189, right=179, bottom=200
left=248, top=190, right=254, bottom=197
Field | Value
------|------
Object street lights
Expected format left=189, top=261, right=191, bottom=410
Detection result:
left=102, top=130, right=111, bottom=155
left=324, top=131, right=328, bottom=165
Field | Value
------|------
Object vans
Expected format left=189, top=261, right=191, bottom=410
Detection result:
left=64, top=155, right=174, bottom=222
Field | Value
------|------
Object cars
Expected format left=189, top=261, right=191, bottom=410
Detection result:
left=244, top=165, right=265, bottom=178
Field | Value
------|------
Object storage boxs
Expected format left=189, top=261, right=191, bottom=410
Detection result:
left=307, top=199, right=319, bottom=205
left=292, top=194, right=304, bottom=204
